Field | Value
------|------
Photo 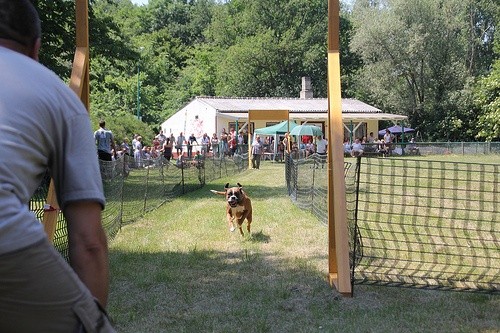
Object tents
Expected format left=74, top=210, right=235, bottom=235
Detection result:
left=253, top=120, right=302, bottom=164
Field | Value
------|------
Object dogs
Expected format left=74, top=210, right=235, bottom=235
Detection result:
left=208, top=181, right=253, bottom=240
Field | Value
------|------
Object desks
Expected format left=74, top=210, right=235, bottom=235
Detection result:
left=261, top=152, right=283, bottom=163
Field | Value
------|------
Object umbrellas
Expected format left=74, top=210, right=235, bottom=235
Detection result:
left=290, top=125, right=323, bottom=150
left=379, top=126, right=414, bottom=142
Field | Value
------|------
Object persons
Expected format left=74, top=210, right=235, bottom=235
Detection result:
left=93, top=121, right=421, bottom=182
left=0, top=0, right=115, bottom=333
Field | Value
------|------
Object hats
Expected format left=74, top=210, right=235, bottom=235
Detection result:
left=0, top=0, right=42, bottom=46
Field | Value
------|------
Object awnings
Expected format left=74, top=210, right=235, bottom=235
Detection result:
left=217, top=113, right=408, bottom=143
